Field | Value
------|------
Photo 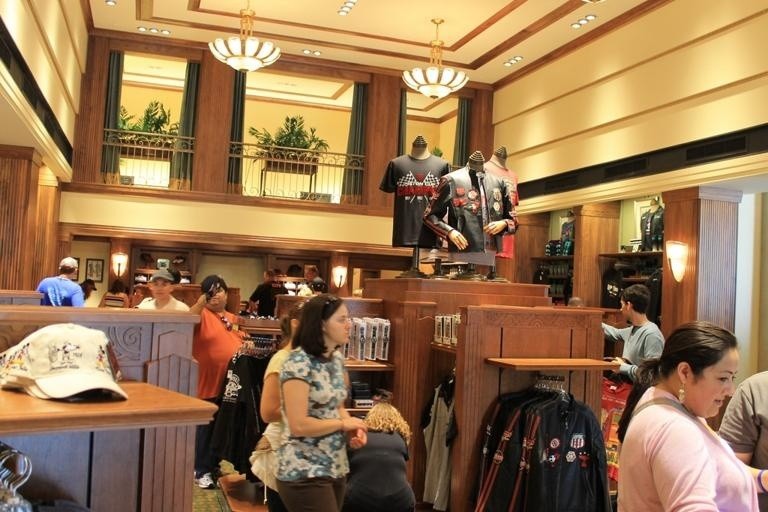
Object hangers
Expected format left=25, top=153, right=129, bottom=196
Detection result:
left=0, top=440, right=90, bottom=510
left=529, top=370, right=578, bottom=408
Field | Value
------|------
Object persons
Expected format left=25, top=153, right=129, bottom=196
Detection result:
left=99, top=278, right=129, bottom=308
left=378, top=135, right=452, bottom=247
left=36, top=257, right=85, bottom=307
left=78, top=279, right=97, bottom=300
left=274, top=296, right=368, bottom=511
left=249, top=269, right=290, bottom=316
left=134, top=269, right=189, bottom=312
left=560, top=211, right=575, bottom=241
left=168, top=266, right=181, bottom=282
left=422, top=150, right=518, bottom=252
left=617, top=322, right=768, bottom=511
left=640, top=200, right=664, bottom=252
left=484, top=147, right=520, bottom=259
left=601, top=285, right=665, bottom=383
left=723, top=369, right=767, bottom=509
left=248, top=297, right=306, bottom=512
left=188, top=274, right=247, bottom=488
left=342, top=401, right=416, bottom=511
left=311, top=277, right=324, bottom=296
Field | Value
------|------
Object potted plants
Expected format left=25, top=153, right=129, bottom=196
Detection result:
left=116, top=99, right=180, bottom=161
left=248, top=112, right=331, bottom=176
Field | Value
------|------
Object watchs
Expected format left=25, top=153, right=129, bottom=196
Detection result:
left=342, top=317, right=391, bottom=361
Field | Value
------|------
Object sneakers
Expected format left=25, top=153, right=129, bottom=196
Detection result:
left=193, top=471, right=215, bottom=489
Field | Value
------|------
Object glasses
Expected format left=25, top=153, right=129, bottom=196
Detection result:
left=221, top=316, right=233, bottom=331
left=323, top=296, right=338, bottom=308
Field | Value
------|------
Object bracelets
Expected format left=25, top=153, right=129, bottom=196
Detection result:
left=756, top=467, right=768, bottom=495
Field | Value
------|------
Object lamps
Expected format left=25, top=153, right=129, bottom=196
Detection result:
left=664, top=238, right=690, bottom=284
left=337, top=0, right=356, bottom=17
left=331, top=263, right=349, bottom=293
left=110, top=251, right=130, bottom=287
left=301, top=49, right=323, bottom=57
left=400, top=15, right=470, bottom=101
left=208, top=0, right=285, bottom=77
left=503, top=55, right=520, bottom=68
left=572, top=14, right=595, bottom=32
left=137, top=25, right=170, bottom=37
left=105, top=0, right=118, bottom=8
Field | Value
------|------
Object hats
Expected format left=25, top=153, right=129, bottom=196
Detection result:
left=0, top=323, right=127, bottom=400
left=84, top=279, right=97, bottom=292
left=150, top=269, right=174, bottom=281
left=59, top=257, right=79, bottom=268
